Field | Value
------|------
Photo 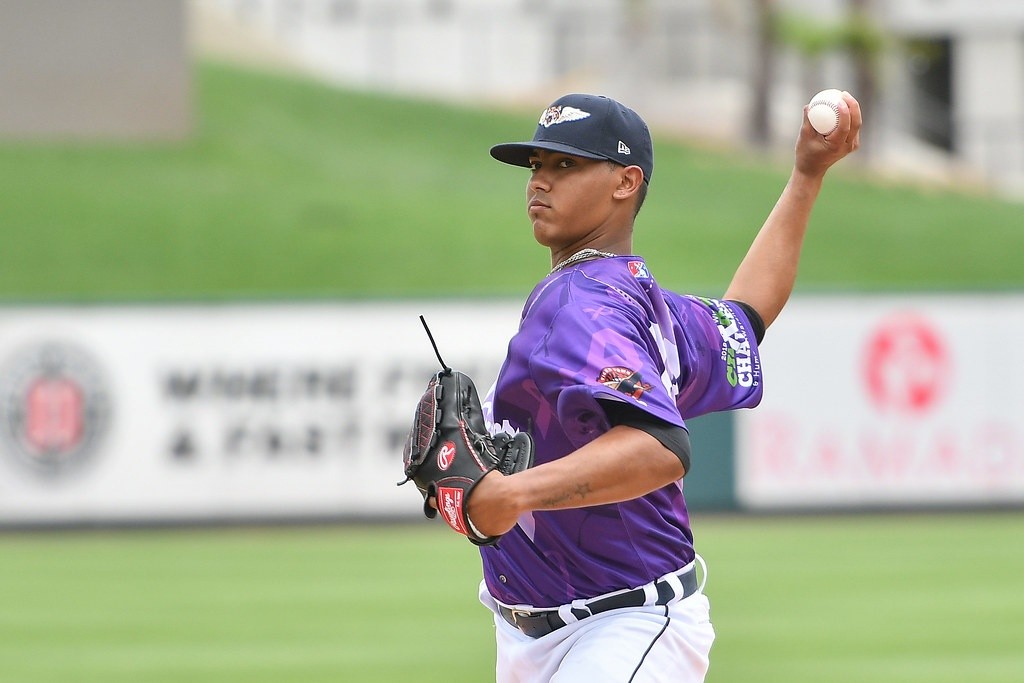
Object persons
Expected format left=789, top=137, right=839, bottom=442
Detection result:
left=404, top=91, right=861, bottom=683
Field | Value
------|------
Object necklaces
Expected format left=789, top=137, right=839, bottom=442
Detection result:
left=546, top=249, right=617, bottom=277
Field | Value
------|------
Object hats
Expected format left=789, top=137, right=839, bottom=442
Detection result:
left=490, top=93, right=654, bottom=184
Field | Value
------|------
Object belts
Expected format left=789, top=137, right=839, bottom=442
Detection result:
left=499, top=566, right=698, bottom=639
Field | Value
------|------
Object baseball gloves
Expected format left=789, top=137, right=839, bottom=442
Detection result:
left=395, top=311, right=538, bottom=551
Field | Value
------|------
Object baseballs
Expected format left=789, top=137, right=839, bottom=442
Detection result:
left=807, top=89, right=848, bottom=136
